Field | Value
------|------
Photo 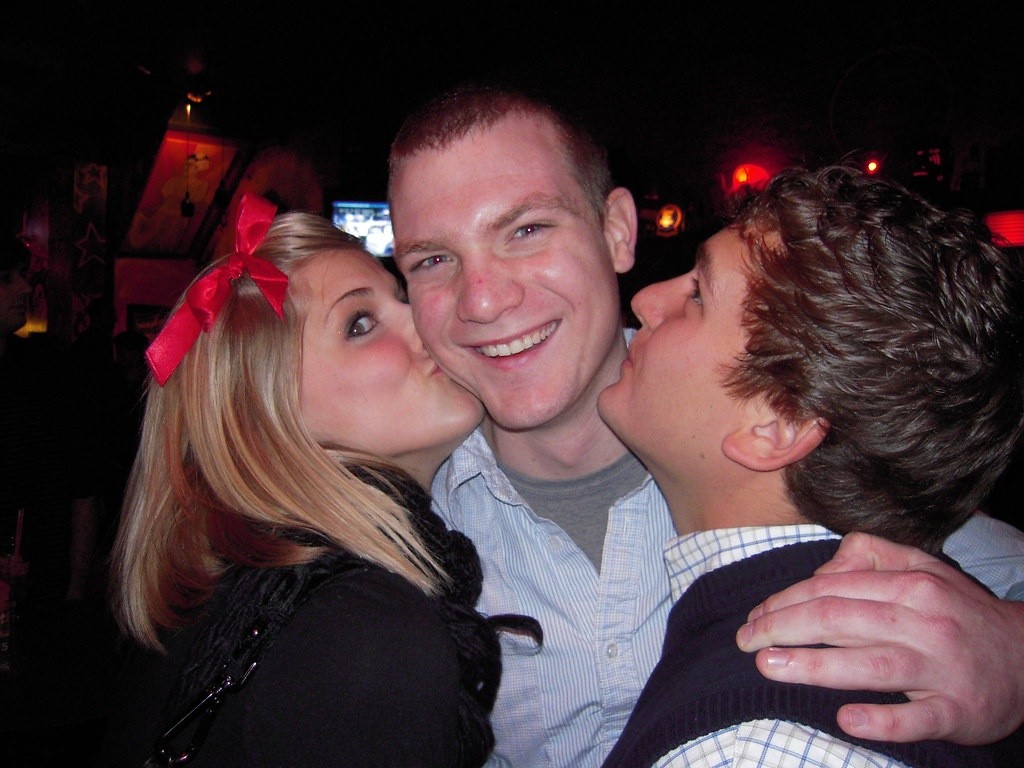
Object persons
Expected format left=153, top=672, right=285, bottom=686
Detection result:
left=108, top=72, right=1024, bottom=768
